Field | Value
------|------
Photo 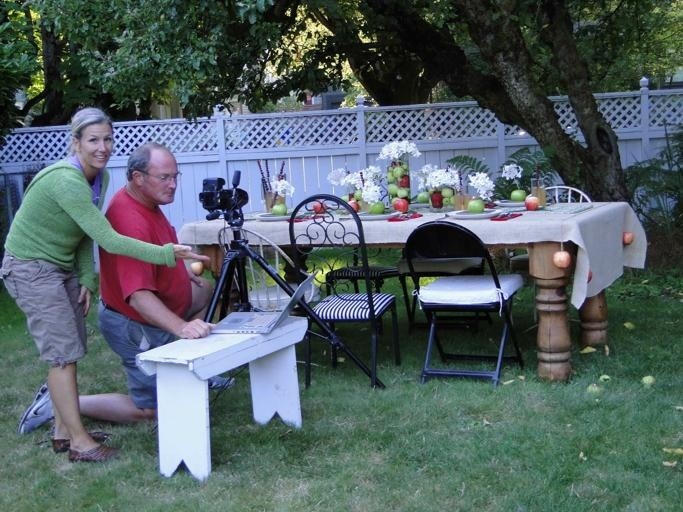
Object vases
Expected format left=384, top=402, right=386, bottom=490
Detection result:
left=348, top=189, right=362, bottom=212
left=417, top=192, right=429, bottom=204
left=387, top=160, right=411, bottom=204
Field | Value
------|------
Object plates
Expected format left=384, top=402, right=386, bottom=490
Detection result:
left=494, top=199, right=527, bottom=207
left=358, top=208, right=402, bottom=221
left=258, top=211, right=304, bottom=221
left=447, top=210, right=500, bottom=219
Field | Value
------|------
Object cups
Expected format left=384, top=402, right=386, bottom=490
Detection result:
left=454, top=184, right=468, bottom=210
left=530, top=178, right=546, bottom=206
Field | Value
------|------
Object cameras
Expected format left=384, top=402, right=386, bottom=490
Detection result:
left=197, top=169, right=250, bottom=225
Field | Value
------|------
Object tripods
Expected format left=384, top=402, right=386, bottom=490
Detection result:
left=204, top=229, right=386, bottom=395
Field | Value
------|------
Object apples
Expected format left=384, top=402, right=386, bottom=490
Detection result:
left=387, top=184, right=398, bottom=195
left=552, top=251, right=571, bottom=268
left=524, top=196, right=539, bottom=211
left=393, top=167, right=405, bottom=178
left=396, top=174, right=409, bottom=187
left=442, top=198, right=450, bottom=205
left=347, top=200, right=360, bottom=213
left=313, top=201, right=327, bottom=214
left=441, top=187, right=453, bottom=197
left=623, top=231, right=633, bottom=244
left=387, top=171, right=396, bottom=183
left=393, top=198, right=409, bottom=213
left=354, top=190, right=362, bottom=201
left=417, top=192, right=429, bottom=203
left=190, top=262, right=204, bottom=277
left=397, top=188, right=409, bottom=199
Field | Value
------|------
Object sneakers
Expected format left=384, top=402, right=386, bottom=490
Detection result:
left=18, top=384, right=55, bottom=437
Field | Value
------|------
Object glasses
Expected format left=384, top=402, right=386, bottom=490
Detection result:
left=134, top=168, right=180, bottom=182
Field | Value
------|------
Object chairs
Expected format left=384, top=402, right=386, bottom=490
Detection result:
left=405, top=221, right=524, bottom=388
left=216, top=226, right=334, bottom=369
left=289, top=193, right=400, bottom=389
left=326, top=248, right=413, bottom=336
left=509, top=186, right=592, bottom=327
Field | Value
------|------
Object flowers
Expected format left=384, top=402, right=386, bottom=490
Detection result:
left=375, top=140, right=422, bottom=161
left=326, top=165, right=382, bottom=189
left=413, top=164, right=438, bottom=192
left=468, top=171, right=495, bottom=201
left=425, top=166, right=461, bottom=192
left=270, top=179, right=295, bottom=203
left=502, top=163, right=523, bottom=190
left=362, top=185, right=381, bottom=206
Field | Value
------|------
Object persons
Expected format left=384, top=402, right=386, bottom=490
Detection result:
left=18, top=143, right=234, bottom=436
left=0, top=107, right=209, bottom=463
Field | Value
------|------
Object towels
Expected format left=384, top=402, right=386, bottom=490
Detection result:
left=491, top=213, right=523, bottom=222
left=387, top=213, right=422, bottom=221
left=287, top=214, right=320, bottom=223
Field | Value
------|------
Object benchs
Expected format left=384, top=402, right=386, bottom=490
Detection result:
left=134, top=315, right=308, bottom=483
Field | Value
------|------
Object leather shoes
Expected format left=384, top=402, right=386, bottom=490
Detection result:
left=52, top=430, right=111, bottom=451
left=69, top=445, right=120, bottom=464
